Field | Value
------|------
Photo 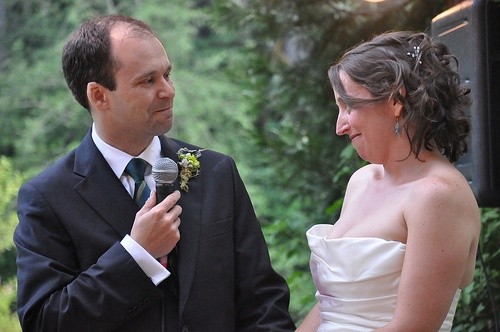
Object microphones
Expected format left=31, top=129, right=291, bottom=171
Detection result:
left=152, top=158, right=179, bottom=256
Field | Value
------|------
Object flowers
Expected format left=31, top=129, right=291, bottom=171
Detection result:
left=177, top=148, right=206, bottom=192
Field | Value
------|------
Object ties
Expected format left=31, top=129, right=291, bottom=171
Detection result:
left=125, top=158, right=150, bottom=209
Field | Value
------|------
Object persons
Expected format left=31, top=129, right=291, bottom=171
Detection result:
left=12, top=14, right=297, bottom=332
left=296, top=30, right=481, bottom=331
